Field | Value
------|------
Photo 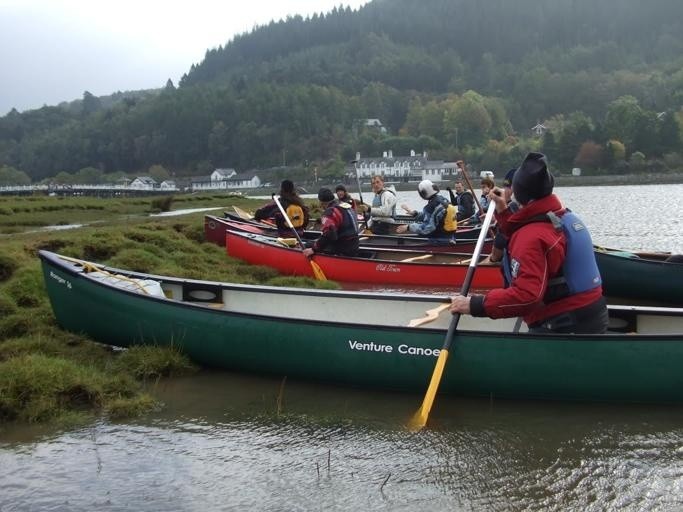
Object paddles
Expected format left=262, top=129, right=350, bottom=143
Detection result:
left=408, top=192, right=502, bottom=431
left=351, top=160, right=373, bottom=234
left=272, top=194, right=328, bottom=281
left=231, top=204, right=277, bottom=228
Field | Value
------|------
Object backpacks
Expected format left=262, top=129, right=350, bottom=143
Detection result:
left=442, top=204, right=458, bottom=232
left=285, top=204, right=305, bottom=228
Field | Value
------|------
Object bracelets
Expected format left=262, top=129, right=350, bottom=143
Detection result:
left=488, top=255, right=496, bottom=262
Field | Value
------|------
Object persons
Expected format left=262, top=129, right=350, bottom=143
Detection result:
left=256, top=180, right=309, bottom=239
left=358, top=175, right=397, bottom=235
left=446, top=181, right=475, bottom=224
left=303, top=188, right=359, bottom=257
left=487, top=166, right=524, bottom=266
left=460, top=177, right=495, bottom=226
left=449, top=152, right=610, bottom=334
left=335, top=185, right=357, bottom=212
left=393, top=180, right=456, bottom=246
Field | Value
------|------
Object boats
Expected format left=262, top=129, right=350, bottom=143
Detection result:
left=223, top=211, right=498, bottom=240
left=231, top=203, right=482, bottom=234
left=223, top=208, right=500, bottom=238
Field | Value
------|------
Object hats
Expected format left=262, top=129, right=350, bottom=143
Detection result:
left=335, top=186, right=347, bottom=192
left=505, top=168, right=518, bottom=185
left=418, top=180, right=440, bottom=200
left=512, top=152, right=554, bottom=207
left=318, top=188, right=335, bottom=203
